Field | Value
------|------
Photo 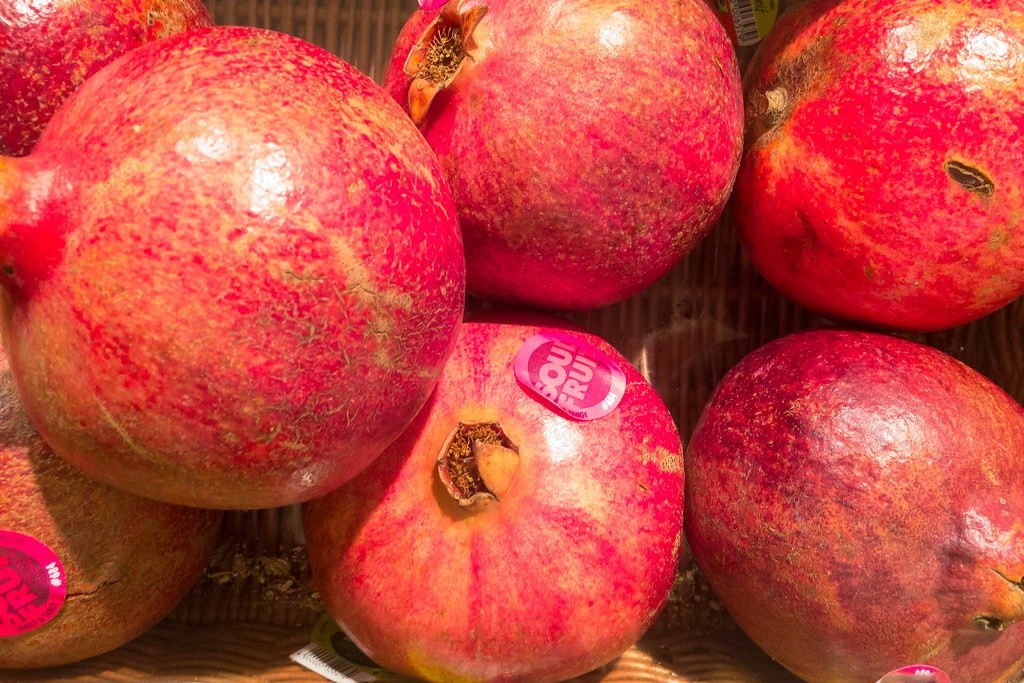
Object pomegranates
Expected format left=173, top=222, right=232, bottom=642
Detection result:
left=0, top=0, right=1024, bottom=683
